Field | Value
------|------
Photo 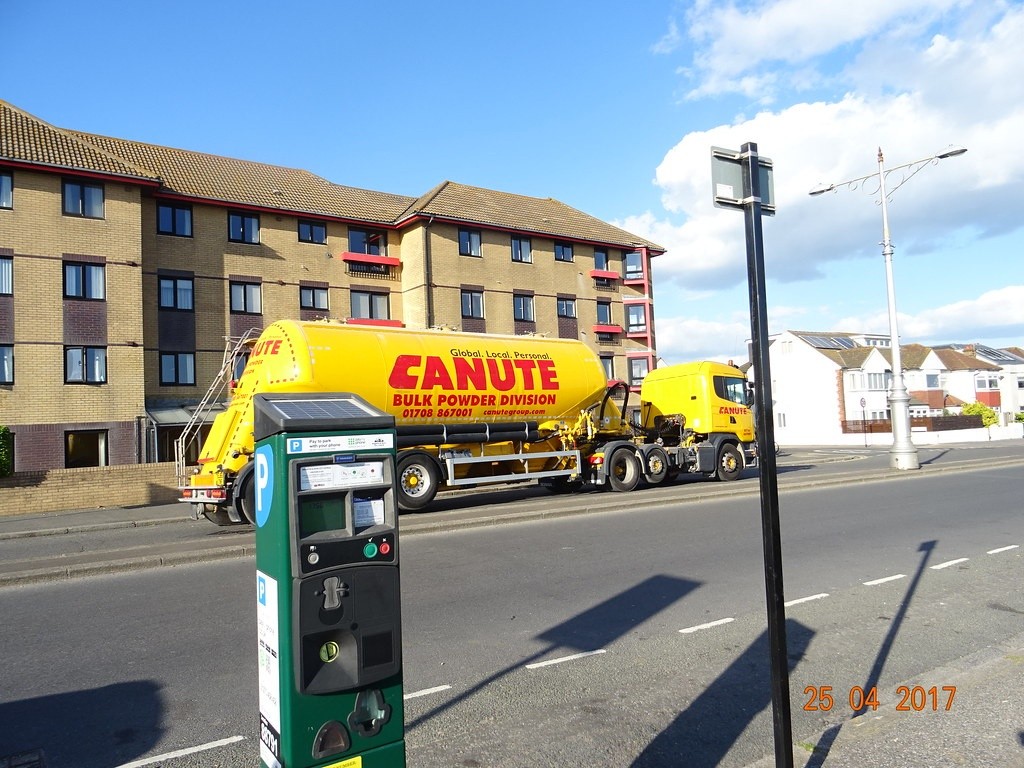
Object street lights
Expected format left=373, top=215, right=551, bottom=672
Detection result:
left=807, top=145, right=969, bottom=471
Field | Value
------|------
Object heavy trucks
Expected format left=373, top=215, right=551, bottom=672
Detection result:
left=174, top=319, right=758, bottom=527
